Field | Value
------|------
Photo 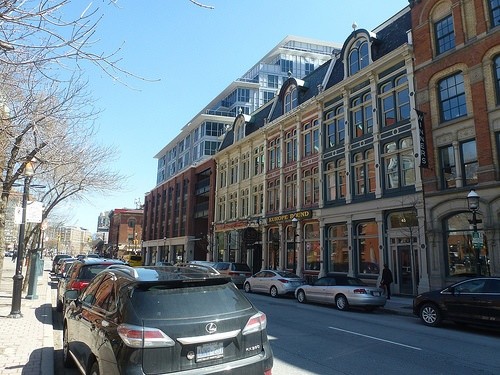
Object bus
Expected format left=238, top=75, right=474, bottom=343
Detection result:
left=120, top=255, right=142, bottom=266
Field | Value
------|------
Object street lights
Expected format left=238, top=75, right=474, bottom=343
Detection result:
left=163, top=236, right=167, bottom=263
left=467, top=191, right=483, bottom=275
left=6, top=163, right=34, bottom=318
left=292, top=218, right=299, bottom=268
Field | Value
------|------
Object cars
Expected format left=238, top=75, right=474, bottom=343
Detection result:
left=51, top=253, right=100, bottom=288
left=243, top=270, right=307, bottom=298
left=413, top=277, right=500, bottom=332
left=306, top=260, right=380, bottom=274
left=5, top=250, right=13, bottom=257
left=294, top=277, right=388, bottom=311
left=402, top=266, right=410, bottom=276
left=209, top=262, right=252, bottom=289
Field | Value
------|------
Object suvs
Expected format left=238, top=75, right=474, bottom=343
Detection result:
left=57, top=257, right=131, bottom=312
left=63, top=263, right=274, bottom=375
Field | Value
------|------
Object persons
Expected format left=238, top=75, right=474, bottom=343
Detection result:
left=380, top=264, right=393, bottom=300
left=12, top=252, right=17, bottom=263
